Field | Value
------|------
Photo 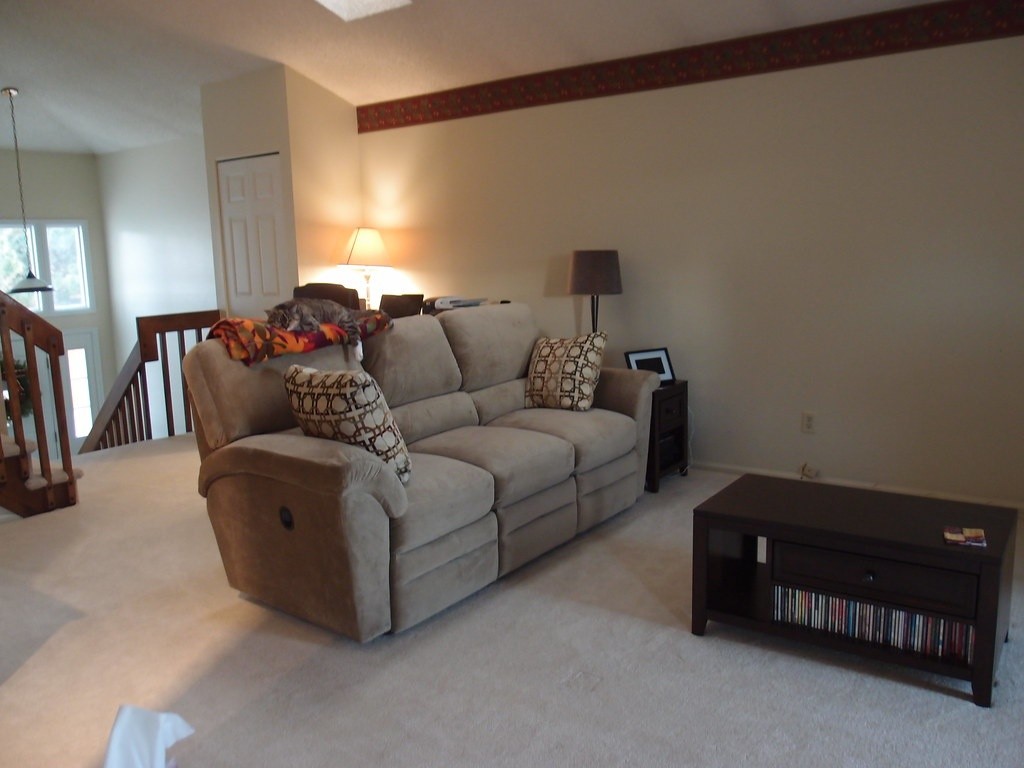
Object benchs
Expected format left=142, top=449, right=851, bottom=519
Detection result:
left=181, top=301, right=661, bottom=645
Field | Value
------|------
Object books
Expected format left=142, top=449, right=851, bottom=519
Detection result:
left=773, top=584, right=976, bottom=668
left=943, top=526, right=987, bottom=548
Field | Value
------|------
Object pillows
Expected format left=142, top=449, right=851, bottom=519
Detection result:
left=284, top=364, right=413, bottom=482
left=525, top=330, right=608, bottom=412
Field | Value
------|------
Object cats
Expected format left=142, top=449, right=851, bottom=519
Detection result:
left=264, top=297, right=395, bottom=362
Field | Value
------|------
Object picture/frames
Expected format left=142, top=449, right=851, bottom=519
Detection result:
left=624, top=347, right=677, bottom=387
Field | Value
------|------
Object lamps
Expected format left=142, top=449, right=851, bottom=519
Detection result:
left=1, top=87, right=55, bottom=294
left=339, top=226, right=392, bottom=310
left=566, top=250, right=623, bottom=334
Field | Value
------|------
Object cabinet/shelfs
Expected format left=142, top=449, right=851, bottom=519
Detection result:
left=691, top=473, right=1019, bottom=708
left=644, top=380, right=689, bottom=493
